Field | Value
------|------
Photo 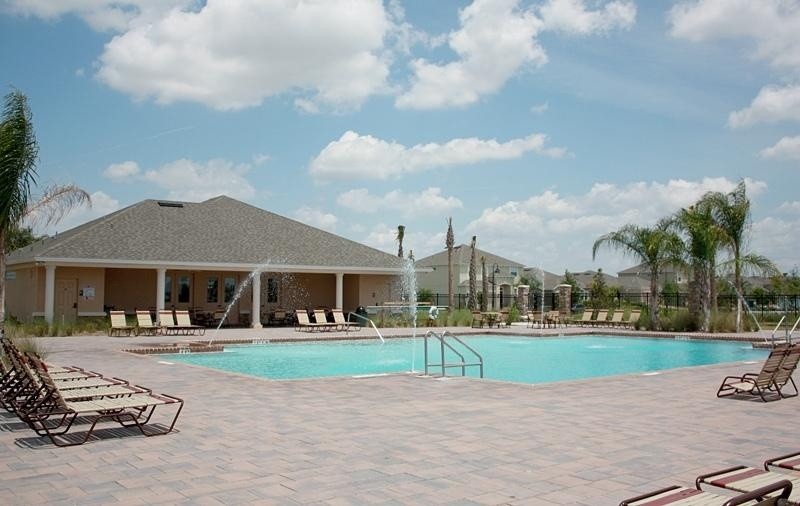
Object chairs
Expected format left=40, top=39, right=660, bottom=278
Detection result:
left=0, top=305, right=230, bottom=452
left=468, top=306, right=642, bottom=331
left=619, top=343, right=800, bottom=506
left=260, top=307, right=362, bottom=334
